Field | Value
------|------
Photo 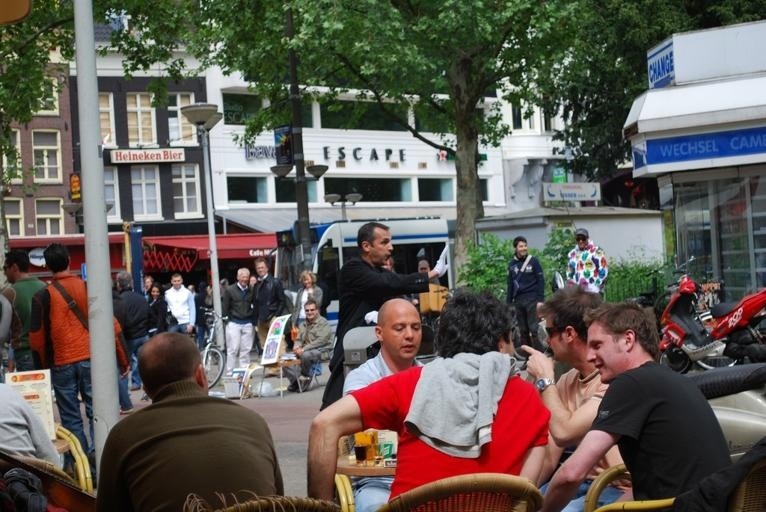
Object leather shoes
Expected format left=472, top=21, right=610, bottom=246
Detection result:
left=287, top=380, right=298, bottom=392
left=297, top=375, right=312, bottom=393
left=130, top=386, right=140, bottom=391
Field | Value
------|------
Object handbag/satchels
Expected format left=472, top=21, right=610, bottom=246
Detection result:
left=291, top=324, right=299, bottom=341
left=165, top=309, right=177, bottom=326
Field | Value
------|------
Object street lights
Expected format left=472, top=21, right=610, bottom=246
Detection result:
left=271, top=163, right=328, bottom=279
left=182, top=102, right=231, bottom=392
left=323, top=192, right=362, bottom=219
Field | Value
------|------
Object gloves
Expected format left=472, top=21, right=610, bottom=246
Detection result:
left=434, top=244, right=450, bottom=279
left=364, top=310, right=378, bottom=325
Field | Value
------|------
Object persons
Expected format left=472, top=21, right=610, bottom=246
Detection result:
left=566, top=227, right=608, bottom=294
left=382, top=256, right=394, bottom=272
left=305, top=287, right=551, bottom=512
left=341, top=297, right=426, bottom=512
left=418, top=259, right=440, bottom=285
left=536, top=285, right=632, bottom=512
left=319, top=221, right=450, bottom=412
left=540, top=299, right=734, bottom=511
left=505, top=235, right=545, bottom=354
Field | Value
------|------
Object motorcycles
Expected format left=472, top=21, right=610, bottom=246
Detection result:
left=627, top=250, right=765, bottom=466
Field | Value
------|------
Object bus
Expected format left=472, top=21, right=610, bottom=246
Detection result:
left=267, top=216, right=451, bottom=341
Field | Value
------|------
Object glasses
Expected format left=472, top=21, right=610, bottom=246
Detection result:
left=304, top=308, right=316, bottom=312
left=576, top=238, right=586, bottom=241
left=545, top=325, right=562, bottom=338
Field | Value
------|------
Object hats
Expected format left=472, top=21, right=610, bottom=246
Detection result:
left=575, top=227, right=589, bottom=239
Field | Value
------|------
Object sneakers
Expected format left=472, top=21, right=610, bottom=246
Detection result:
left=119, top=408, right=132, bottom=414
left=140, top=392, right=148, bottom=401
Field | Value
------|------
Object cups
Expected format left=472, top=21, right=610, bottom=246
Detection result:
left=353, top=429, right=393, bottom=467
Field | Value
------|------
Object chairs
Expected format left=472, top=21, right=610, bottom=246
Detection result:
left=180, top=489, right=343, bottom=512
left=9, top=454, right=79, bottom=488
left=300, top=299, right=339, bottom=392
left=584, top=456, right=766, bottom=512
left=332, top=434, right=360, bottom=512
left=375, top=473, right=545, bottom=512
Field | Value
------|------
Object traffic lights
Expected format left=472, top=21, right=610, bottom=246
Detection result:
left=70, top=173, right=81, bottom=202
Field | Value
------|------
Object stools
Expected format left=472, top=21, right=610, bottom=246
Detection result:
left=256, top=359, right=303, bottom=400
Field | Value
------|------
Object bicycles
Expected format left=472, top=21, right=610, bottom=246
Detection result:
left=508, top=305, right=532, bottom=362
left=198, top=308, right=230, bottom=389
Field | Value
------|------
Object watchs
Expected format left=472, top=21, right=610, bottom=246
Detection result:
left=534, top=377, right=556, bottom=392
